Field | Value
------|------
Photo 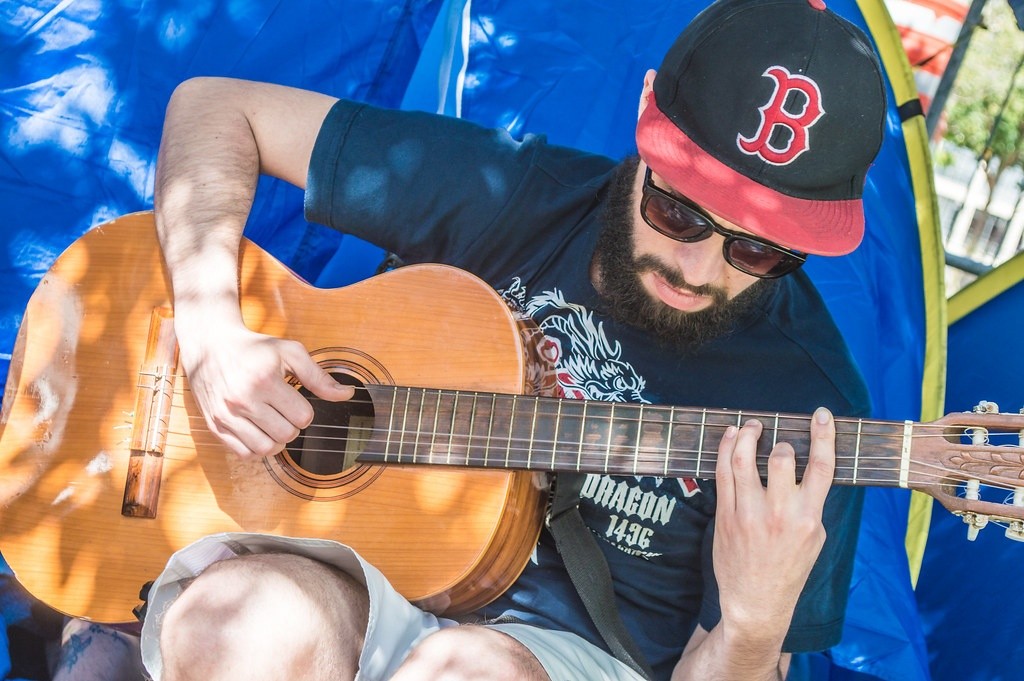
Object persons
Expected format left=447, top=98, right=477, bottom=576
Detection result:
left=140, top=1, right=886, bottom=681
left=54, top=615, right=152, bottom=681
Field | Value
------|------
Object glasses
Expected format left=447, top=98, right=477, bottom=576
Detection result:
left=640, top=165, right=807, bottom=280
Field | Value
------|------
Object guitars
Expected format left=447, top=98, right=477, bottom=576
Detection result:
left=0, top=206, right=1024, bottom=628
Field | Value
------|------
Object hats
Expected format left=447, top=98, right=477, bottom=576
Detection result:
left=636, top=0, right=886, bottom=258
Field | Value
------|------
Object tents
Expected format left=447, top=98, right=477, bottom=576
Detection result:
left=1, top=0, right=946, bottom=681
left=914, top=249, right=1024, bottom=681
